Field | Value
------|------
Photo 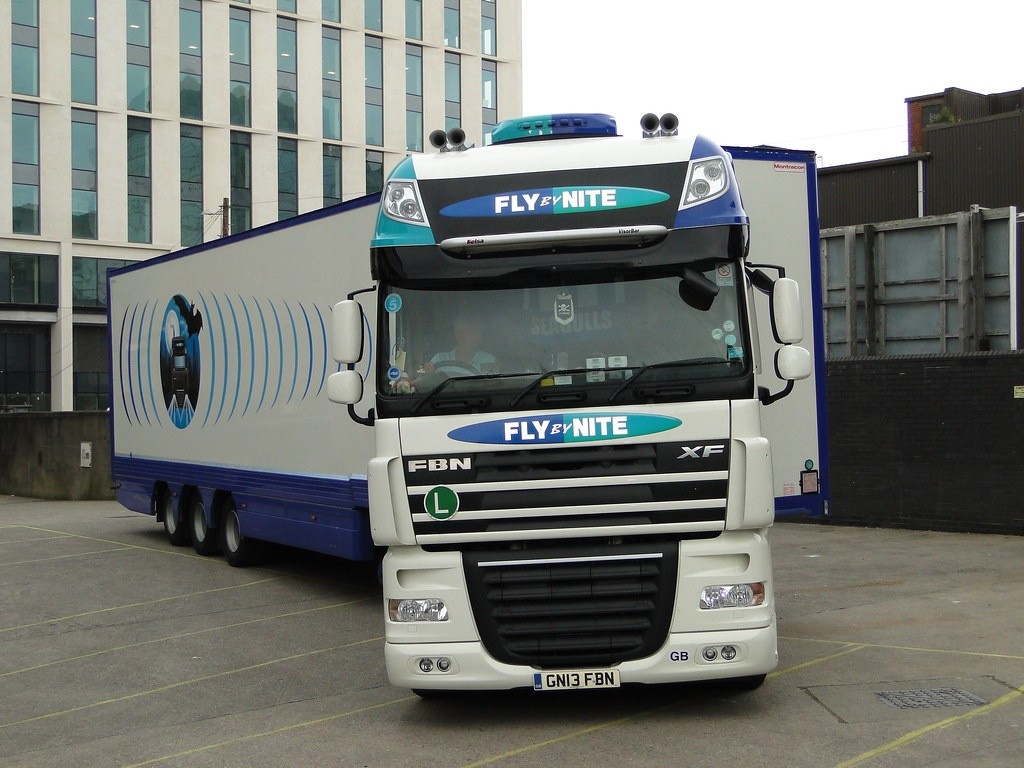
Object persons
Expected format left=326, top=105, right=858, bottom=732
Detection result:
left=422, top=310, right=502, bottom=378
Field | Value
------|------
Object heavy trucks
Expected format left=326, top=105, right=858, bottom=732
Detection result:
left=107, top=111, right=818, bottom=713
